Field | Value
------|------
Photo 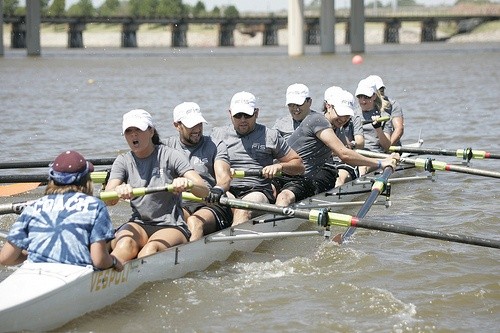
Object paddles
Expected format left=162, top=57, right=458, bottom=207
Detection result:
left=182, top=191, right=500, bottom=249
left=361, top=116, right=390, bottom=125
left=328, top=159, right=398, bottom=246
left=0, top=158, right=116, bottom=169
left=353, top=149, right=500, bottom=179
left=389, top=146, right=500, bottom=160
left=0, top=170, right=282, bottom=183
left=0, top=179, right=194, bottom=216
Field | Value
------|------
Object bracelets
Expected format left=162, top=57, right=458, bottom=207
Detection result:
left=378, top=160, right=381, bottom=168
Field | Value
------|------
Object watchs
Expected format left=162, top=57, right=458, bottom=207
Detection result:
left=108, top=256, right=117, bottom=269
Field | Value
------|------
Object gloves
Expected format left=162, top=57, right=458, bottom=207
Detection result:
left=202, top=187, right=225, bottom=204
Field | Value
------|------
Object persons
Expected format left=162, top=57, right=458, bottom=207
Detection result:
left=0, top=150, right=122, bottom=270
left=98, top=109, right=210, bottom=264
left=350, top=79, right=392, bottom=178
left=271, top=82, right=324, bottom=163
left=366, top=75, right=404, bottom=157
left=210, top=91, right=305, bottom=228
left=270, top=86, right=401, bottom=207
left=161, top=101, right=235, bottom=241
left=312, top=86, right=365, bottom=187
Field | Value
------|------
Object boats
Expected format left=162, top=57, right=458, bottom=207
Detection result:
left=0, top=139, right=424, bottom=333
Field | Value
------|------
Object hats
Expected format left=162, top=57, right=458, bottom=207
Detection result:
left=230, top=91, right=256, bottom=116
left=331, top=90, right=355, bottom=116
left=173, top=101, right=209, bottom=128
left=122, top=108, right=154, bottom=135
left=354, top=86, right=374, bottom=98
left=358, top=79, right=378, bottom=91
left=285, top=83, right=311, bottom=106
left=366, top=75, right=386, bottom=91
left=52, top=151, right=94, bottom=174
left=325, top=86, right=342, bottom=102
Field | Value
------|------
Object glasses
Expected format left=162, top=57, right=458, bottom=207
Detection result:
left=233, top=111, right=256, bottom=119
